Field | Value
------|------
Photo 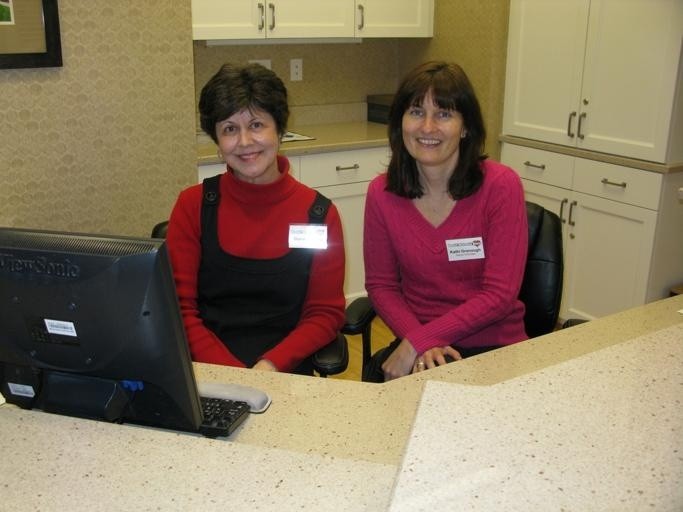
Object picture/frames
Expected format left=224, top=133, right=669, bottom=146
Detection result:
left=0, top=0, right=62, bottom=71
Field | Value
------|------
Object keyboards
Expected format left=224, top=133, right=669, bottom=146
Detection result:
left=199, top=397, right=250, bottom=437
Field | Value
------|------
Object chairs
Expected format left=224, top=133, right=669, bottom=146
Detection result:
left=342, top=199, right=588, bottom=380
left=150, top=199, right=351, bottom=382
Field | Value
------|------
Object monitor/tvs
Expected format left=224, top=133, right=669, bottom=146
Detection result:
left=0, top=228, right=204, bottom=431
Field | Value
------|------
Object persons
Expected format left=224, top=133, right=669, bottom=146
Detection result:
left=362, top=62, right=528, bottom=383
left=166, top=60, right=346, bottom=372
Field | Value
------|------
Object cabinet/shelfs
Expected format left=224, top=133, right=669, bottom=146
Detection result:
left=190, top=1, right=354, bottom=40
left=499, top=0, right=683, bottom=165
left=499, top=143, right=683, bottom=327
left=198, top=146, right=393, bottom=311
left=354, top=0, right=434, bottom=39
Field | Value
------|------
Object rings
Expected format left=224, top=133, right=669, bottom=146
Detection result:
left=417, top=362, right=425, bottom=365
